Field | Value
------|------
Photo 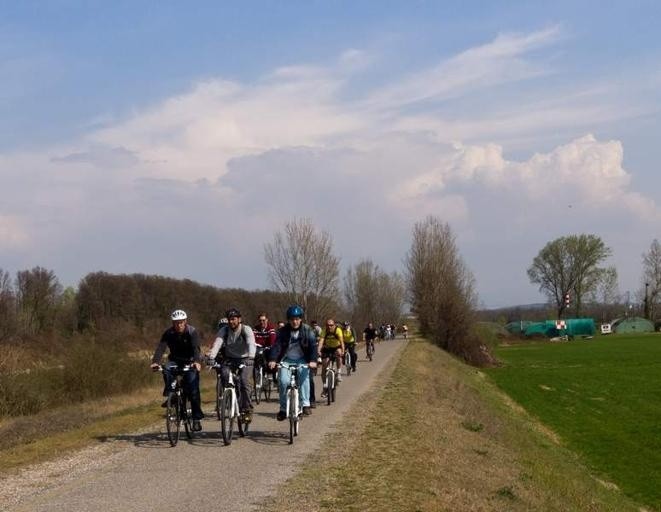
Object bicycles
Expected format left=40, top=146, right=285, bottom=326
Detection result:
left=205, top=329, right=409, bottom=421
left=207, top=360, right=255, bottom=446
left=266, top=360, right=323, bottom=443
left=153, top=362, right=201, bottom=447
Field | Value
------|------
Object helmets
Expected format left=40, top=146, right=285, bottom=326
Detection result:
left=218, top=309, right=239, bottom=327
left=170, top=309, right=187, bottom=321
left=286, top=305, right=303, bottom=319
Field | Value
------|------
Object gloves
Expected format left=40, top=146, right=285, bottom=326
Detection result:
left=244, top=357, right=253, bottom=365
left=206, top=359, right=215, bottom=365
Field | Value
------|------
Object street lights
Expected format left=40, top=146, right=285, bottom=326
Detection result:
left=645, top=283, right=650, bottom=317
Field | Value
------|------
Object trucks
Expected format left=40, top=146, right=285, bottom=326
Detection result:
left=601, top=324, right=613, bottom=335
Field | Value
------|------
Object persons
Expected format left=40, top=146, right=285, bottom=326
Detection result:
left=252, top=312, right=277, bottom=391
left=149, top=308, right=206, bottom=432
left=312, top=317, right=409, bottom=398
left=207, top=306, right=257, bottom=425
left=218, top=317, right=229, bottom=328
left=268, top=304, right=318, bottom=421
left=277, top=320, right=286, bottom=330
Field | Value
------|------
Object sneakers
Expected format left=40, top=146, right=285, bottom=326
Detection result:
left=193, top=421, right=202, bottom=431
left=277, top=410, right=286, bottom=420
left=304, top=402, right=315, bottom=415
